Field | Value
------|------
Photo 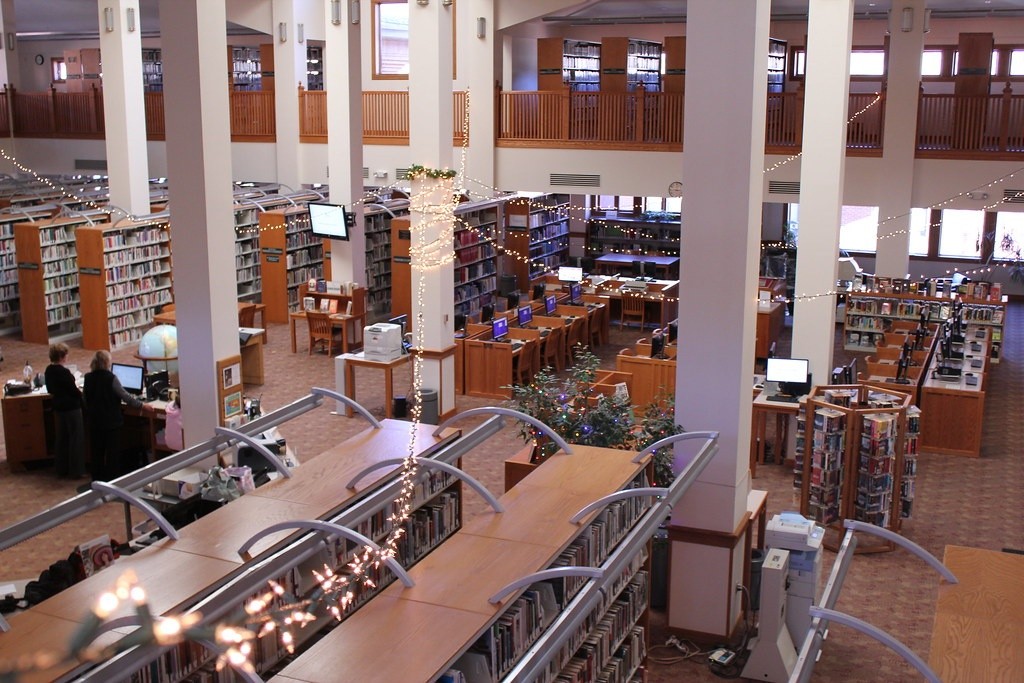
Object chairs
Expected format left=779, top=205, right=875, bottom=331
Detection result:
left=304, top=312, right=342, bottom=357
left=450, top=284, right=645, bottom=387
left=239, top=304, right=255, bottom=330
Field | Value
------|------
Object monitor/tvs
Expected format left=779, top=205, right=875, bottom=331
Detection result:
left=307, top=202, right=349, bottom=241
left=455, top=257, right=592, bottom=343
left=843, top=358, right=857, bottom=384
left=651, top=318, right=678, bottom=360
left=894, top=297, right=967, bottom=384
left=633, top=261, right=656, bottom=282
left=766, top=358, right=809, bottom=385
left=389, top=315, right=408, bottom=336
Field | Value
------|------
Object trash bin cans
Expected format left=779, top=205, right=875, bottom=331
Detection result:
left=413, top=388, right=438, bottom=424
left=751, top=548, right=765, bottom=610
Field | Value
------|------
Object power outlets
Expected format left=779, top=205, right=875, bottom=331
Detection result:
left=735, top=580, right=741, bottom=594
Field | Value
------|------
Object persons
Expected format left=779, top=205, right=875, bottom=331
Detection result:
left=82, top=349, right=153, bottom=472
left=165, top=388, right=184, bottom=455
left=44, top=342, right=84, bottom=461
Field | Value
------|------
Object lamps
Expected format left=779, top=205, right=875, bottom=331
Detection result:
left=476, top=16, right=486, bottom=38
left=280, top=23, right=303, bottom=43
left=902, top=8, right=931, bottom=32
left=331, top=0, right=361, bottom=25
left=105, top=8, right=135, bottom=31
left=8, top=33, right=14, bottom=50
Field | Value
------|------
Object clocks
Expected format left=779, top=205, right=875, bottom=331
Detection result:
left=35, top=55, right=44, bottom=65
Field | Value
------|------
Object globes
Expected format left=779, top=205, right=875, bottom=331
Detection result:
left=138, top=325, right=179, bottom=374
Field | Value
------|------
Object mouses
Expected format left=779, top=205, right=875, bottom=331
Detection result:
left=522, top=339, right=526, bottom=342
left=546, top=327, right=551, bottom=329
left=595, top=302, right=600, bottom=304
left=570, top=315, right=575, bottom=318
left=970, top=341, right=976, bottom=344
left=979, top=328, right=984, bottom=330
left=965, top=373, right=972, bottom=376
left=966, top=355, right=973, bottom=358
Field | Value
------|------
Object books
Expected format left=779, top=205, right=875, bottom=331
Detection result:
left=790, top=388, right=921, bottom=528
left=845, top=274, right=1003, bottom=357
left=0, top=175, right=680, bottom=350
left=307, top=46, right=322, bottom=89
left=78, top=534, right=115, bottom=578
left=563, top=46, right=600, bottom=118
left=142, top=49, right=163, bottom=91
left=232, top=46, right=261, bottom=91
left=768, top=44, right=785, bottom=92
left=628, top=43, right=661, bottom=111
left=121, top=461, right=653, bottom=682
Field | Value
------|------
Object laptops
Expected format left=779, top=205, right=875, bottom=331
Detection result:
left=111, top=362, right=144, bottom=396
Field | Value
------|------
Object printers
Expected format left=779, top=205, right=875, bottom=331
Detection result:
left=763, top=512, right=826, bottom=598
left=364, top=322, right=401, bottom=362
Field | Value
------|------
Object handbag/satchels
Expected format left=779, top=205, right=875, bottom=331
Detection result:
left=224, top=465, right=255, bottom=494
left=200, top=464, right=243, bottom=502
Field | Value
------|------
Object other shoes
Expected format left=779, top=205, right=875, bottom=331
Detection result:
left=56, top=472, right=65, bottom=478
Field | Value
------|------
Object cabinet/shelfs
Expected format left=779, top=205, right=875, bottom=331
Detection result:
left=766, top=37, right=789, bottom=145
left=0, top=174, right=571, bottom=344
left=585, top=217, right=679, bottom=277
left=662, top=36, right=687, bottom=141
left=953, top=31, right=995, bottom=149
left=227, top=46, right=262, bottom=91
left=308, top=46, right=323, bottom=91
left=599, top=37, right=665, bottom=140
left=142, top=47, right=162, bottom=90
left=537, top=37, right=600, bottom=139
left=0, top=416, right=657, bottom=683
left=843, top=292, right=1005, bottom=363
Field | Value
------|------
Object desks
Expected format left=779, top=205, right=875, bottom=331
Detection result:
left=155, top=254, right=995, bottom=491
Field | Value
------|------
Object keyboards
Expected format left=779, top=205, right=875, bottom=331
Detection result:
left=565, top=319, right=573, bottom=325
left=586, top=305, right=596, bottom=311
left=965, top=377, right=977, bottom=385
left=766, top=395, right=800, bottom=403
left=971, top=359, right=982, bottom=368
left=975, top=331, right=985, bottom=338
left=512, top=343, right=523, bottom=350
left=540, top=330, right=549, bottom=337
left=971, top=345, right=981, bottom=351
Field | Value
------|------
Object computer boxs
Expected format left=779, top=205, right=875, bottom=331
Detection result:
left=144, top=370, right=168, bottom=401
left=832, top=367, right=844, bottom=385
left=783, top=373, right=812, bottom=395
